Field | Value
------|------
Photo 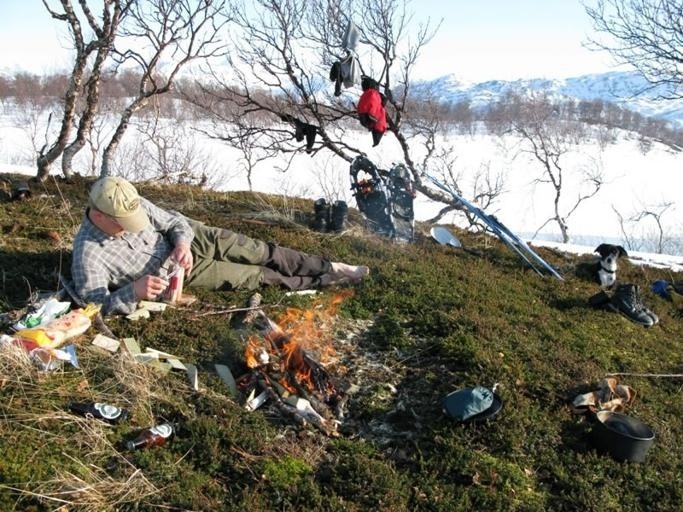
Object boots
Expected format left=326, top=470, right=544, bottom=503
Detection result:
left=331, top=200, right=348, bottom=231
left=609, top=284, right=659, bottom=326
left=309, top=198, right=331, bottom=233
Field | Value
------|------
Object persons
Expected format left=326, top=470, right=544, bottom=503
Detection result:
left=71, top=177, right=370, bottom=315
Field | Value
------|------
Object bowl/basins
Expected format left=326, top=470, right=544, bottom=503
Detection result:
left=596, top=409, right=657, bottom=464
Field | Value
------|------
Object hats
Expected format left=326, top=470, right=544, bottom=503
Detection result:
left=89, top=175, right=150, bottom=233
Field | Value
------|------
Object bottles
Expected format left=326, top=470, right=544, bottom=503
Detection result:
left=67, top=398, right=131, bottom=425
left=124, top=421, right=173, bottom=452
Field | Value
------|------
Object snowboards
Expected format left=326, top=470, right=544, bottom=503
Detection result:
left=351, top=156, right=414, bottom=245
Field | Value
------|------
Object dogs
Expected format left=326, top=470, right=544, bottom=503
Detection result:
left=575, top=244, right=627, bottom=288
